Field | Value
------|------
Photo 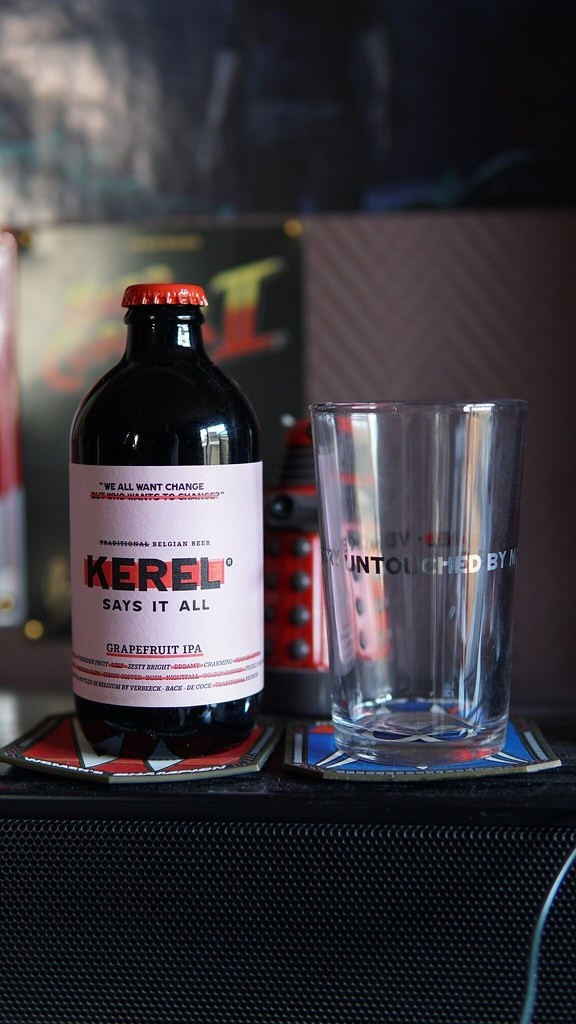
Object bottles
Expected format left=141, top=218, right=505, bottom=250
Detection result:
left=64, top=283, right=267, bottom=758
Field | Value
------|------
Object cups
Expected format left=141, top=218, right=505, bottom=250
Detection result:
left=309, top=398, right=526, bottom=763
